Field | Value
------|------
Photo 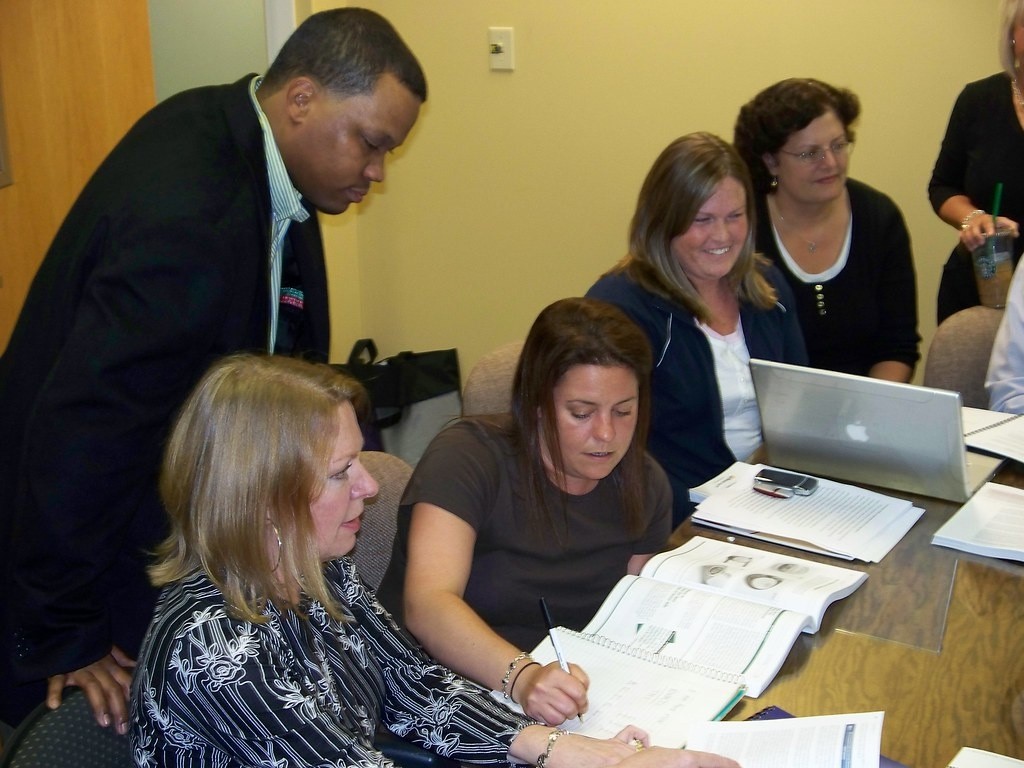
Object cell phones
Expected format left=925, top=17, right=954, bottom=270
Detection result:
left=755, top=470, right=819, bottom=496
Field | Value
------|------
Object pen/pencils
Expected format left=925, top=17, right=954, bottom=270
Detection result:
left=540, top=597, right=584, bottom=723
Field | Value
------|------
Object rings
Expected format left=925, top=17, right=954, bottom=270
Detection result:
left=635, top=739, right=643, bottom=752
left=961, top=224, right=970, bottom=232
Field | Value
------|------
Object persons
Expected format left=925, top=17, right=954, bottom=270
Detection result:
left=984, top=252, right=1024, bottom=418
left=730, top=77, right=923, bottom=384
left=0, top=6, right=428, bottom=736
left=927, top=0, right=1024, bottom=326
left=583, top=131, right=811, bottom=533
left=128, top=352, right=742, bottom=768
left=373, top=297, right=672, bottom=693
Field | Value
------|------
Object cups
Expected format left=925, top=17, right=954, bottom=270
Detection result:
left=971, top=228, right=1015, bottom=308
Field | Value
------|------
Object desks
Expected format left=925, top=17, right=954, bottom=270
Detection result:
left=667, top=442, right=1024, bottom=768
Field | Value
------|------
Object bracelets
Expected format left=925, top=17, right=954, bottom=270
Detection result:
left=510, top=662, right=541, bottom=704
left=536, top=730, right=569, bottom=768
left=502, top=652, right=532, bottom=699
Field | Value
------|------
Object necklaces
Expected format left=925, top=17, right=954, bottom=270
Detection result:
left=772, top=195, right=831, bottom=253
left=1012, top=79, right=1024, bottom=107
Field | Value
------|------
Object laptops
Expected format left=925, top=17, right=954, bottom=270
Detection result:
left=747, top=358, right=1009, bottom=502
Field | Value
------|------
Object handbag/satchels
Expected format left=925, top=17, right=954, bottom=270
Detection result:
left=331, top=339, right=466, bottom=466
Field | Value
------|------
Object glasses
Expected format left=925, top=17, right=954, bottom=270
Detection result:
left=772, top=140, right=853, bottom=165
